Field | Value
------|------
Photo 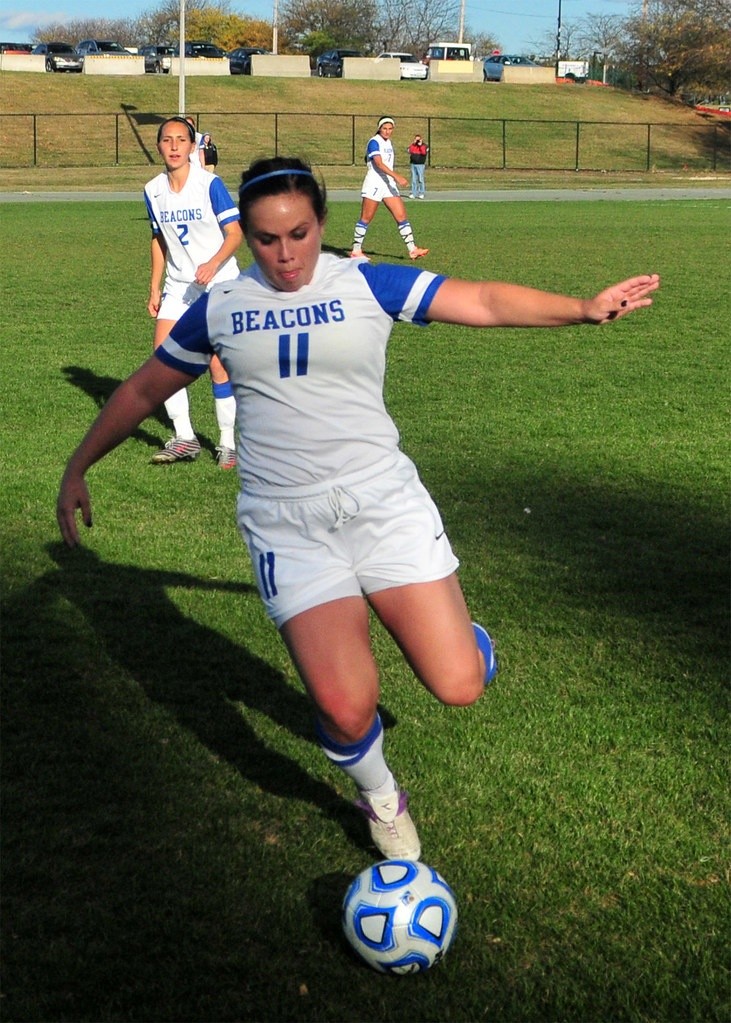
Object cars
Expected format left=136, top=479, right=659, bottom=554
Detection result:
left=226, top=47, right=272, bottom=74
left=78, top=39, right=134, bottom=55
left=138, top=46, right=178, bottom=74
left=317, top=49, right=368, bottom=77
left=376, top=52, right=429, bottom=80
left=31, top=42, right=84, bottom=73
left=480, top=55, right=541, bottom=82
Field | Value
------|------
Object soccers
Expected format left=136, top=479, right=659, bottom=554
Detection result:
left=342, top=859, right=458, bottom=978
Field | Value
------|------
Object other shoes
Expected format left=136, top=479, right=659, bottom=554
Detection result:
left=419, top=196, right=424, bottom=199
left=409, top=194, right=415, bottom=199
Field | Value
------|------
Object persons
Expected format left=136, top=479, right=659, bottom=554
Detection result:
left=202, top=133, right=219, bottom=174
left=184, top=115, right=207, bottom=172
left=54, top=155, right=661, bottom=862
left=143, top=117, right=246, bottom=470
left=406, top=134, right=429, bottom=199
left=350, top=116, right=430, bottom=266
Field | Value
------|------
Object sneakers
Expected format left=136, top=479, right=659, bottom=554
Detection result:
left=350, top=251, right=370, bottom=262
left=214, top=445, right=236, bottom=469
left=409, top=247, right=429, bottom=260
left=356, top=775, right=421, bottom=864
left=152, top=435, right=201, bottom=464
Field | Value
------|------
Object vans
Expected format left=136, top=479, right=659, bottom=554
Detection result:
left=423, top=41, right=472, bottom=68
left=172, top=41, right=225, bottom=58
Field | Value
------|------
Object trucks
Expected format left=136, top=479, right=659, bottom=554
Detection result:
left=554, top=60, right=590, bottom=84
